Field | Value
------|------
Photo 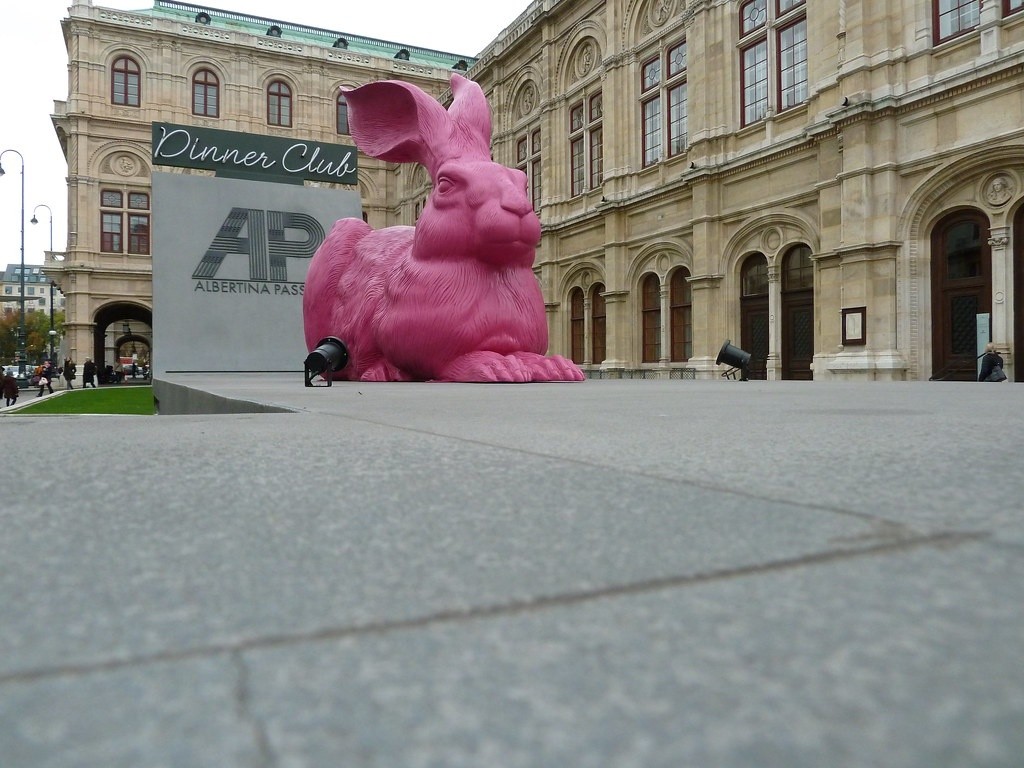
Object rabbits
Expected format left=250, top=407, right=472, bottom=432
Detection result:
left=300, top=71, right=584, bottom=386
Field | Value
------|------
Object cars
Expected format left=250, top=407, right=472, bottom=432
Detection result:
left=122, top=363, right=142, bottom=375
left=2, top=364, right=39, bottom=379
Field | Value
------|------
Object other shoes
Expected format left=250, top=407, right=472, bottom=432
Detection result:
left=36, top=394, right=41, bottom=397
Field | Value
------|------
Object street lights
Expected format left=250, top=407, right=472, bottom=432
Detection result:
left=0, top=150, right=29, bottom=389
left=30, top=204, right=56, bottom=379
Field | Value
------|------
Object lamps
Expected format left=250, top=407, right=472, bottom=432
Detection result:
left=715, top=339, right=753, bottom=383
left=303, top=336, right=349, bottom=387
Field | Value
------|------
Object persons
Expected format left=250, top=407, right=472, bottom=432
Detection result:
left=0, top=356, right=149, bottom=407
left=979, top=343, right=1004, bottom=382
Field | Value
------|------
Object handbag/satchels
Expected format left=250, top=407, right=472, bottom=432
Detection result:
left=984, top=366, right=1007, bottom=382
left=72, top=375, right=76, bottom=379
left=34, top=376, right=40, bottom=382
left=38, top=377, right=48, bottom=385
